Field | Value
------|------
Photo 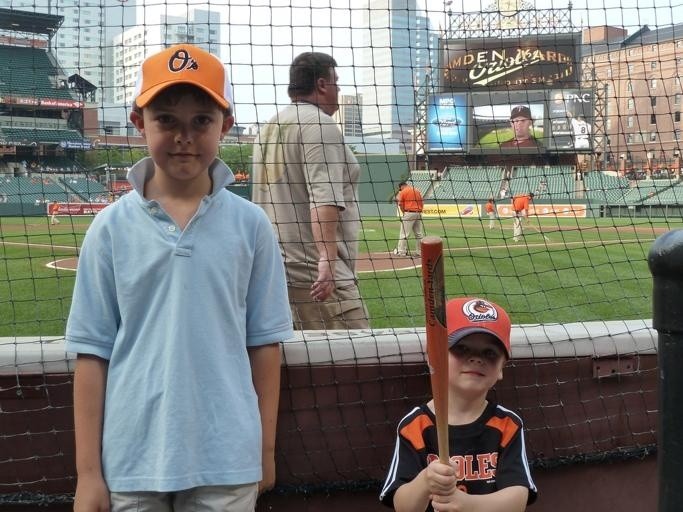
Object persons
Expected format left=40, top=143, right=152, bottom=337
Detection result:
left=392, top=181, right=426, bottom=257
left=0, top=157, right=250, bottom=208
left=564, top=109, right=592, bottom=149
left=64, top=43, right=294, bottom=511
left=508, top=192, right=533, bottom=243
left=50, top=200, right=60, bottom=228
left=483, top=198, right=497, bottom=230
left=498, top=104, right=541, bottom=147
left=595, top=159, right=682, bottom=181
left=247, top=51, right=370, bottom=329
left=377, top=296, right=538, bottom=512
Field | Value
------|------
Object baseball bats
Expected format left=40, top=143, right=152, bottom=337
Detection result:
left=419, top=235, right=450, bottom=465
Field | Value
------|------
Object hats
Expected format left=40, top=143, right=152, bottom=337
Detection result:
left=510, top=105, right=533, bottom=122
left=133, top=42, right=237, bottom=119
left=445, top=297, right=512, bottom=361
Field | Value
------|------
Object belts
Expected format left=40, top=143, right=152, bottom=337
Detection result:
left=404, top=210, right=423, bottom=213
